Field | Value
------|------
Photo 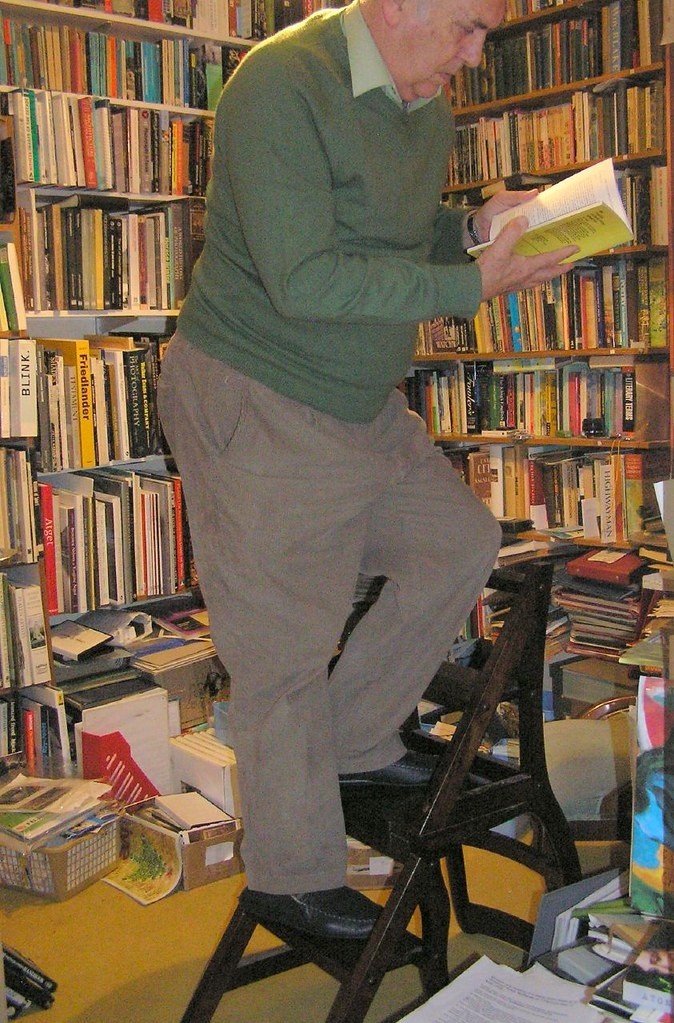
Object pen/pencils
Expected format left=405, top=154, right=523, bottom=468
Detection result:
left=5, top=973, right=53, bottom=1010
left=6, top=1000, right=23, bottom=1019
left=3, top=958, right=56, bottom=1003
left=3, top=951, right=59, bottom=992
left=5, top=985, right=32, bottom=1010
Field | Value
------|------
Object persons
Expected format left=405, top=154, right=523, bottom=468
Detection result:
left=634, top=944, right=674, bottom=976
left=157, top=0, right=580, bottom=936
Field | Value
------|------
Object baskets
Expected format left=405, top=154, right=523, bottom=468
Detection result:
left=0, top=819, right=119, bottom=903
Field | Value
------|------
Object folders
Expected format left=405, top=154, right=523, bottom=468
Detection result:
left=124, top=635, right=187, bottom=659
left=566, top=550, right=650, bottom=585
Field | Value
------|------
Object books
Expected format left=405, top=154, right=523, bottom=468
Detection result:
left=0, top=0, right=349, bottom=904
left=532, top=868, right=674, bottom=1023
left=467, top=159, right=635, bottom=265
left=394, top=0, right=674, bottom=840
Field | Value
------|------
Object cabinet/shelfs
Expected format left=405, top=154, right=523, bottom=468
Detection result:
left=4, top=0, right=673, bottom=780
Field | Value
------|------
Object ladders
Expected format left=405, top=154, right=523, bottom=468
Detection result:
left=181, top=561, right=583, bottom=1023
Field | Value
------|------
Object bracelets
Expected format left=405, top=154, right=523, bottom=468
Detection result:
left=467, top=211, right=483, bottom=246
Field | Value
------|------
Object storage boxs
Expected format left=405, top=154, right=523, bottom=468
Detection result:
left=138, top=656, right=232, bottom=728
left=120, top=794, right=244, bottom=890
left=346, top=844, right=402, bottom=893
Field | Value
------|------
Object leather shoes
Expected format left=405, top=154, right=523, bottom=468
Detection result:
left=239, top=885, right=385, bottom=938
left=338, top=750, right=471, bottom=791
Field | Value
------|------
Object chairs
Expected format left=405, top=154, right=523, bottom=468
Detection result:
left=539, top=696, right=637, bottom=888
